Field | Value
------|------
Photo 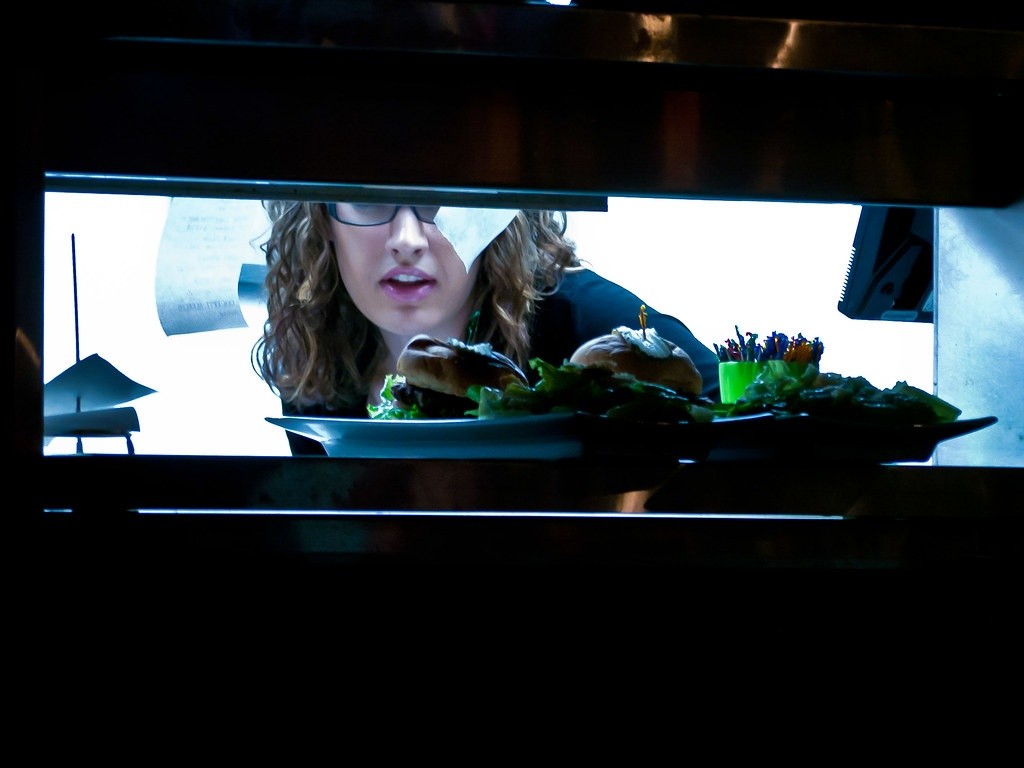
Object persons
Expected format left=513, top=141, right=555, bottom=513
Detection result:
left=250, top=200, right=718, bottom=456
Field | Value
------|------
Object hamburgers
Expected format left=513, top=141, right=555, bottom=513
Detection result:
left=366, top=334, right=529, bottom=420
left=566, top=327, right=702, bottom=397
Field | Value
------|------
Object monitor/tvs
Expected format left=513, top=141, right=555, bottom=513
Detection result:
left=836, top=206, right=935, bottom=324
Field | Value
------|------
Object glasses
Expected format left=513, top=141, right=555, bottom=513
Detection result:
left=324, top=202, right=440, bottom=226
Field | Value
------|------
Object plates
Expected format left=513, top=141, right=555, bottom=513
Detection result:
left=266, top=413, right=611, bottom=460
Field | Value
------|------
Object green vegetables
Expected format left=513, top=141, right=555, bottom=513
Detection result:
left=468, top=356, right=962, bottom=426
